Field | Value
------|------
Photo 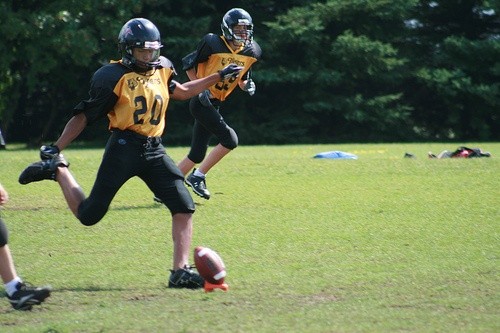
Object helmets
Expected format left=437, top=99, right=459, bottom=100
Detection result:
left=221, top=8, right=253, bottom=42
left=117, top=18, right=162, bottom=76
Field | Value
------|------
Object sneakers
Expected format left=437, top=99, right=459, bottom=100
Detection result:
left=169, top=267, right=204, bottom=288
left=185, top=168, right=211, bottom=201
left=5, top=281, right=49, bottom=312
left=19, top=157, right=69, bottom=185
left=155, top=197, right=163, bottom=203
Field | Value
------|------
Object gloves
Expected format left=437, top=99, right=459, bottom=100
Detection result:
left=41, top=145, right=60, bottom=161
left=199, top=90, right=214, bottom=107
left=245, top=79, right=255, bottom=96
left=218, top=63, right=244, bottom=83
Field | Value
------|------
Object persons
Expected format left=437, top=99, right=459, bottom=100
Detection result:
left=18, top=18, right=246, bottom=289
left=0, top=181, right=51, bottom=312
left=153, top=8, right=261, bottom=203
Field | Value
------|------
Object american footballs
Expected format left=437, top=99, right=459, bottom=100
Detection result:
left=193, top=245, right=225, bottom=285
